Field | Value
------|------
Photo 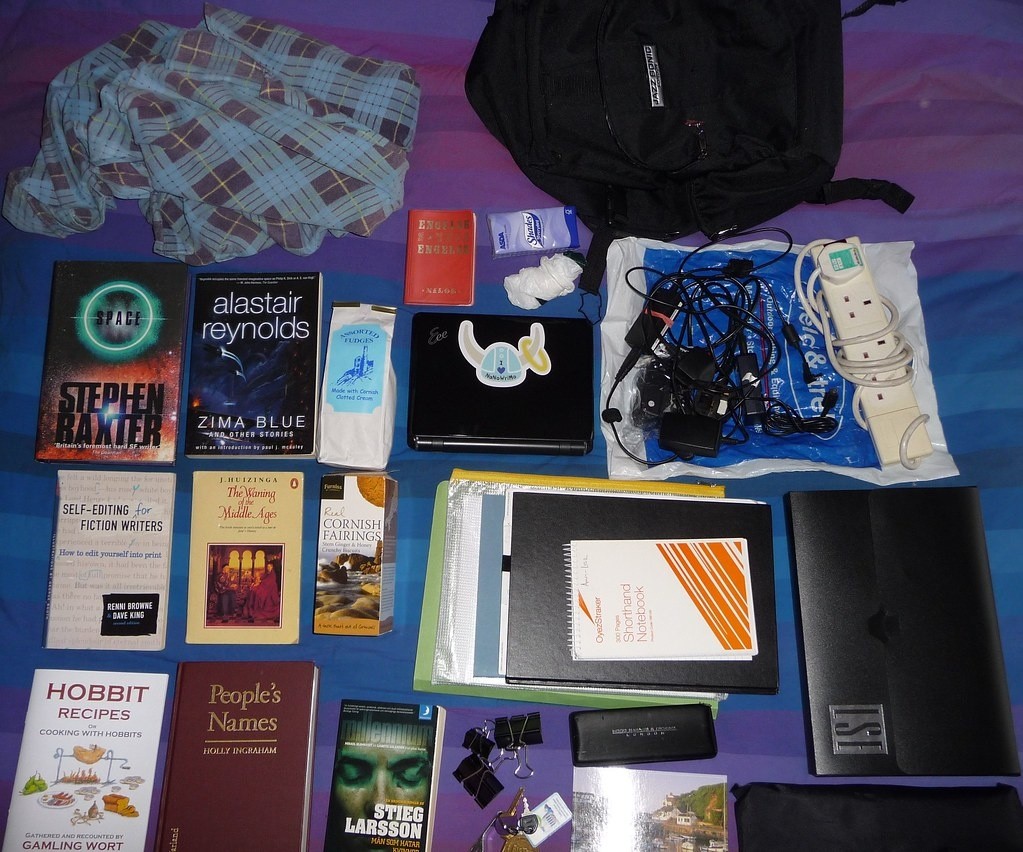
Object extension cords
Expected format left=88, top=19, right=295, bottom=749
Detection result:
left=812, top=236, right=934, bottom=468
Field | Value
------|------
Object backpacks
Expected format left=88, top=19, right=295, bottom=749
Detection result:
left=462, top=0, right=844, bottom=240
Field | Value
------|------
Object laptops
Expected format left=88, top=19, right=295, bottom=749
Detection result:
left=407, top=312, right=595, bottom=457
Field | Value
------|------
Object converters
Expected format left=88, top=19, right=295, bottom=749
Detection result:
left=625, top=288, right=684, bottom=354
left=733, top=353, right=766, bottom=425
left=659, top=412, right=723, bottom=458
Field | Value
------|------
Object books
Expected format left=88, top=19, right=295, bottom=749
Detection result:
left=0, top=207, right=1021, bottom=852
